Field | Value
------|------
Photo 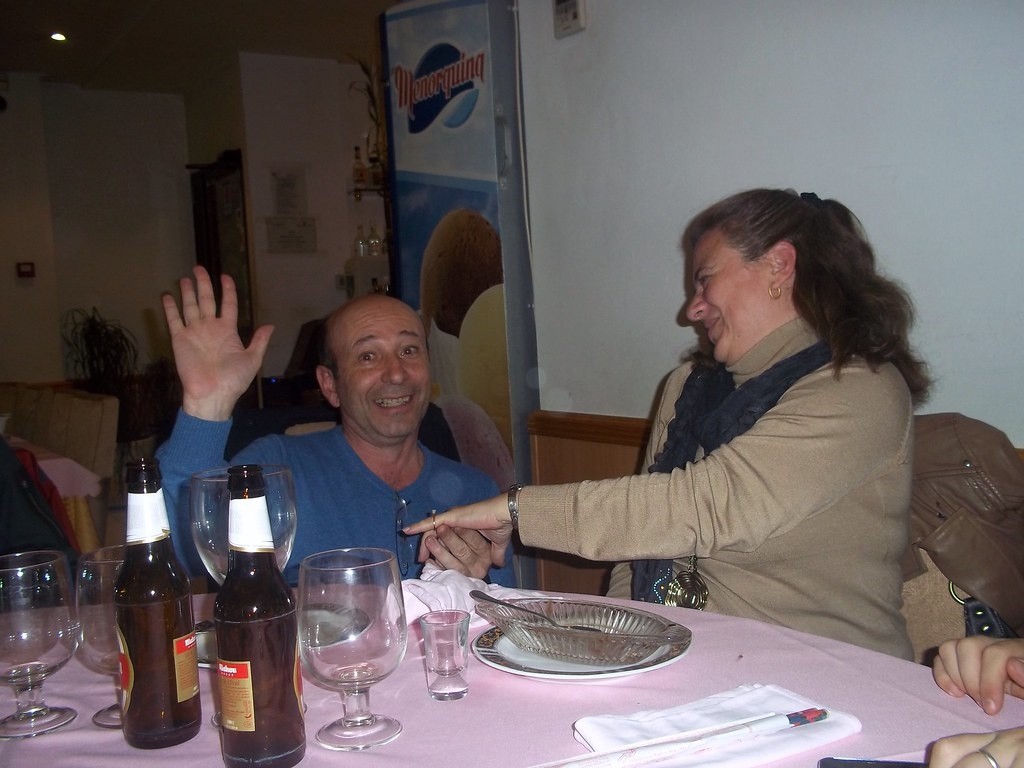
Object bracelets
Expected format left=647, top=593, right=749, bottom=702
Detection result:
left=508, top=483, right=521, bottom=530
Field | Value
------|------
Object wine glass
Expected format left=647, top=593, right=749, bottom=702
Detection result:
left=0, top=549, right=79, bottom=740
left=73, top=545, right=124, bottom=729
left=190, top=463, right=308, bottom=729
left=297, top=547, right=408, bottom=749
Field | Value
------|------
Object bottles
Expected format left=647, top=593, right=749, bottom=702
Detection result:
left=368, top=224, right=383, bottom=255
left=213, top=465, right=307, bottom=767
left=354, top=225, right=369, bottom=257
left=113, top=457, right=202, bottom=749
left=352, top=147, right=366, bottom=189
left=367, top=151, right=383, bottom=189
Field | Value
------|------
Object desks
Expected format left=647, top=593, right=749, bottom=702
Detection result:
left=0, top=587, right=1023, bottom=768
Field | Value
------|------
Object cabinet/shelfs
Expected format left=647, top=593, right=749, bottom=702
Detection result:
left=352, top=189, right=385, bottom=263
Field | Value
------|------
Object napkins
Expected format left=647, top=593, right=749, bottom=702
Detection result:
left=381, top=565, right=564, bottom=630
left=575, top=684, right=863, bottom=768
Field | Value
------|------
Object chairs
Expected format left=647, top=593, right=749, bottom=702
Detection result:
left=899, top=412, right=1024, bottom=669
left=0, top=384, right=120, bottom=547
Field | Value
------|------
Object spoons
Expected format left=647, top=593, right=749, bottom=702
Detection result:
left=469, top=590, right=602, bottom=632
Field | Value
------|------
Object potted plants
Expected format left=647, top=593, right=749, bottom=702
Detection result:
left=60, top=306, right=156, bottom=508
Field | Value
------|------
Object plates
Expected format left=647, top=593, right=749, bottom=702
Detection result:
left=471, top=625, right=692, bottom=684
left=193, top=602, right=373, bottom=669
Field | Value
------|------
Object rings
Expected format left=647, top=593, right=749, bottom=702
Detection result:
left=431, top=509, right=437, bottom=529
left=979, top=750, right=999, bottom=768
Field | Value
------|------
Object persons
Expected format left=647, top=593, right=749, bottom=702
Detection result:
left=403, top=188, right=932, bottom=661
left=154, top=265, right=518, bottom=590
left=929, top=635, right=1024, bottom=768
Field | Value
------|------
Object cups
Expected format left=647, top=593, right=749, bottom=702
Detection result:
left=419, top=610, right=470, bottom=701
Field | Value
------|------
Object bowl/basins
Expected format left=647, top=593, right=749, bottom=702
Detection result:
left=474, top=598, right=693, bottom=666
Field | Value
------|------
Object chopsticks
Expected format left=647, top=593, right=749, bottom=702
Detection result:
left=786, top=707, right=830, bottom=727
